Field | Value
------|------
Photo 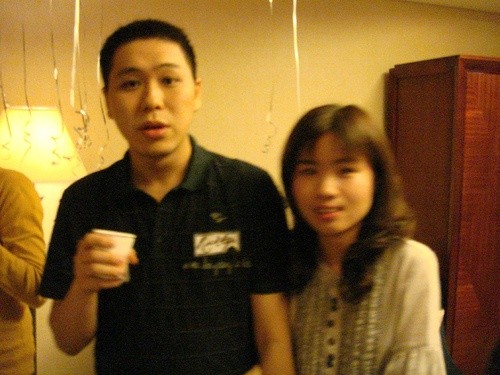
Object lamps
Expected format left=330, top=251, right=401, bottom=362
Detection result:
left=1, top=103, right=90, bottom=185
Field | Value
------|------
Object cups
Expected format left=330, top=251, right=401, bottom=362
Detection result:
left=92, top=229, right=137, bottom=273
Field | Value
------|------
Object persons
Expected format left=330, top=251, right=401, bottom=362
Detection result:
left=36, top=19, right=303, bottom=375
left=0, top=167, right=46, bottom=374
left=239, top=104, right=450, bottom=375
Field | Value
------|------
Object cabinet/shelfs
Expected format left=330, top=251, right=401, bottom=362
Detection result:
left=386, top=53, right=500, bottom=375
